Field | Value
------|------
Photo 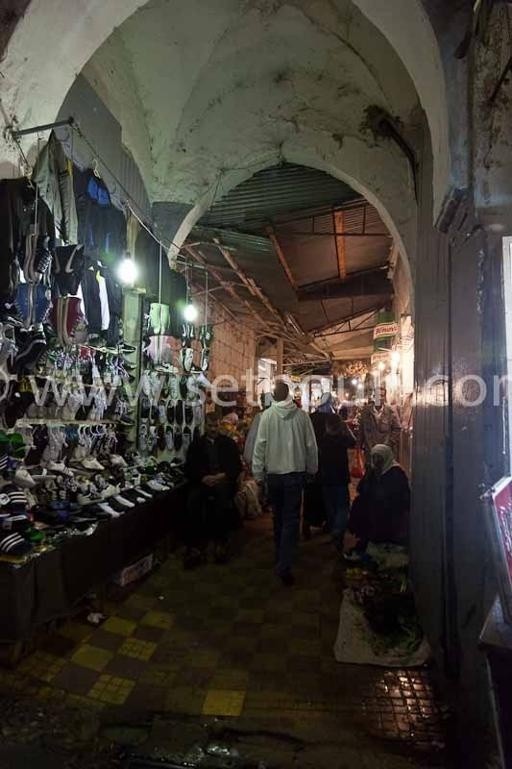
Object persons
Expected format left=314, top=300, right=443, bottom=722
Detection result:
left=242, top=407, right=265, bottom=518
left=250, top=382, right=322, bottom=582
left=301, top=391, right=335, bottom=540
left=343, top=443, right=409, bottom=563
left=182, top=412, right=243, bottom=569
left=356, top=388, right=400, bottom=471
left=315, top=413, right=353, bottom=533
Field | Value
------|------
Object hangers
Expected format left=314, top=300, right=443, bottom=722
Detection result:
left=53, top=126, right=82, bottom=167
left=89, top=157, right=102, bottom=180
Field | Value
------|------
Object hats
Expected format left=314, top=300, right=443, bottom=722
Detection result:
left=317, top=392, right=331, bottom=407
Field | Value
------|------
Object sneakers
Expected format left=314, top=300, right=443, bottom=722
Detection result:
left=342, top=550, right=363, bottom=561
left=12, top=234, right=86, bottom=348
left=184, top=545, right=227, bottom=565
left=276, top=563, right=296, bottom=584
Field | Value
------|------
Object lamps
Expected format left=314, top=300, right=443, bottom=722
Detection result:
left=182, top=263, right=199, bottom=323
left=116, top=204, right=140, bottom=287
left=350, top=351, right=414, bottom=393
